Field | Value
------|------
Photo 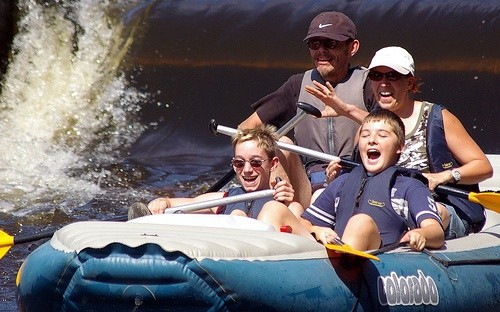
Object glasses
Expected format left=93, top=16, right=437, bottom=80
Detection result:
left=230, top=157, right=272, bottom=168
left=306, top=40, right=345, bottom=51
left=368, top=69, right=406, bottom=81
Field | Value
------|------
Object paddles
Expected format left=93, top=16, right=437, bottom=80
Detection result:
left=324, top=235, right=381, bottom=261
left=206, top=101, right=323, bottom=193
left=0, top=176, right=285, bottom=260
left=217, top=123, right=497, bottom=213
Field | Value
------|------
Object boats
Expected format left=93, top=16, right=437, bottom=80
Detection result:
left=15, top=153, right=500, bottom=312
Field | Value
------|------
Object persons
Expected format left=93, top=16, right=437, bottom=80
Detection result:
left=128, top=11, right=493, bottom=252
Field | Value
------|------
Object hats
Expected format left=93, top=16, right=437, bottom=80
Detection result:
left=303, top=11, right=358, bottom=41
left=365, top=46, right=414, bottom=77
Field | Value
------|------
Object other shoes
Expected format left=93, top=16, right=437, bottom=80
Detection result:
left=128, top=201, right=152, bottom=220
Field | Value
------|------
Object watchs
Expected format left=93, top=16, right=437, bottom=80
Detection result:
left=449, top=168, right=462, bottom=185
